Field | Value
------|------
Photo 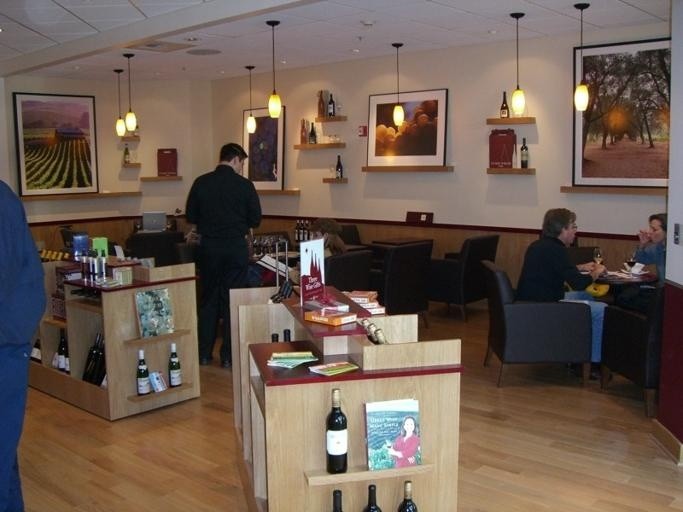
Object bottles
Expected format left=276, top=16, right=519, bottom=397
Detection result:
left=327, top=92, right=335, bottom=116
left=397, top=479, right=417, bottom=512
left=168, top=343, right=182, bottom=386
left=134, top=120, right=139, bottom=137
left=499, top=91, right=510, bottom=118
left=57, top=330, right=69, bottom=373
left=124, top=143, right=130, bottom=164
left=318, top=90, right=324, bottom=115
left=361, top=319, right=387, bottom=344
left=300, top=119, right=307, bottom=144
left=309, top=122, right=316, bottom=144
left=521, top=137, right=528, bottom=168
left=336, top=155, right=342, bottom=177
left=326, top=388, right=348, bottom=473
left=70, top=289, right=101, bottom=299
left=80, top=248, right=107, bottom=276
left=332, top=490, right=343, bottom=512
left=135, top=351, right=151, bottom=395
left=362, top=485, right=381, bottom=512
left=85, top=334, right=106, bottom=384
left=294, top=220, right=315, bottom=243
left=272, top=329, right=291, bottom=343
left=30, top=337, right=41, bottom=363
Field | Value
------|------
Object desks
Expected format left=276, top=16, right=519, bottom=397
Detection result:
left=576, top=267, right=645, bottom=290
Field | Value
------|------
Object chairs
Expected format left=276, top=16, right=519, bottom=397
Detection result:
left=370, top=242, right=432, bottom=331
left=127, top=219, right=187, bottom=263
left=426, top=234, right=494, bottom=320
left=59, top=224, right=117, bottom=256
left=478, top=258, right=590, bottom=390
left=601, top=282, right=665, bottom=418
left=311, top=248, right=366, bottom=300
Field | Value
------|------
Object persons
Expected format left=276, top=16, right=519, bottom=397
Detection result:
left=514, top=207, right=613, bottom=382
left=387, top=416, right=420, bottom=468
left=290, top=219, right=348, bottom=286
left=633, top=213, right=667, bottom=284
left=185, top=142, right=262, bottom=369
left=0, top=179, right=47, bottom=512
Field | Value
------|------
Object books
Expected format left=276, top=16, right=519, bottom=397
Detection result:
left=363, top=399, right=422, bottom=471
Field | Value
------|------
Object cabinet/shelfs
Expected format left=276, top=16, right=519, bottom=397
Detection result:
left=24, top=243, right=197, bottom=421
left=230, top=284, right=461, bottom=511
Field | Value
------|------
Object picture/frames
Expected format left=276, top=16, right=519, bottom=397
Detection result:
left=240, top=107, right=284, bottom=190
left=12, top=92, right=96, bottom=195
left=571, top=38, right=668, bottom=186
left=364, top=90, right=447, bottom=166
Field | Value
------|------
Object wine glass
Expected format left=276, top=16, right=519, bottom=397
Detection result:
left=626, top=252, right=637, bottom=278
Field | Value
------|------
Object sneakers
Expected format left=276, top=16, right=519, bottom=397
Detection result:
left=576, top=372, right=611, bottom=385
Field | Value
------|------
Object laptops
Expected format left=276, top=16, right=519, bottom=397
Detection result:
left=143, top=210, right=167, bottom=229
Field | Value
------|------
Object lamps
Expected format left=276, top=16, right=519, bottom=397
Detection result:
left=572, top=2, right=594, bottom=112
left=242, top=64, right=261, bottom=133
left=264, top=22, right=284, bottom=117
left=114, top=68, right=126, bottom=136
left=509, top=11, right=525, bottom=114
left=120, top=53, right=139, bottom=130
left=391, top=41, right=405, bottom=126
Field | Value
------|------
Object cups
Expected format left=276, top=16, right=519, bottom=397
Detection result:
left=317, top=134, right=341, bottom=143
left=593, top=248, right=604, bottom=264
